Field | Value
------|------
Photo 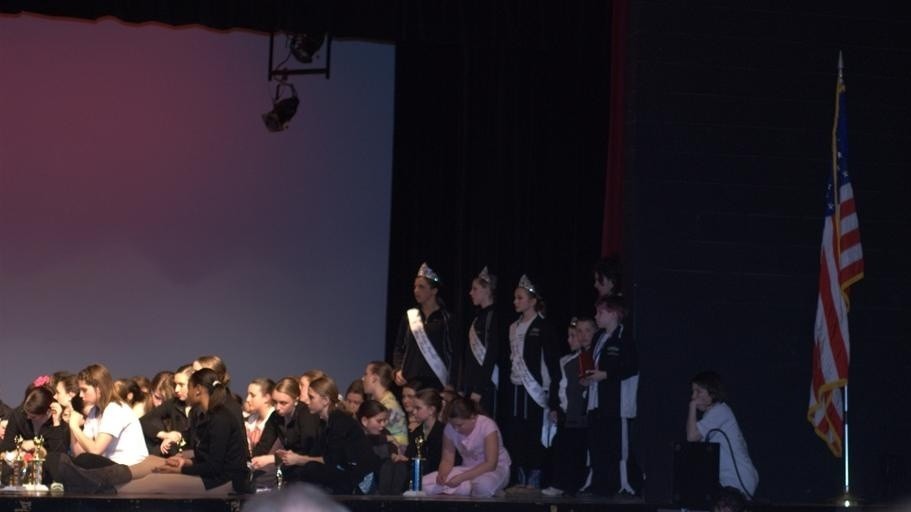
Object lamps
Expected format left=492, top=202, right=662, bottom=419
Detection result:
left=262, top=0, right=326, bottom=66
left=258, top=70, right=300, bottom=130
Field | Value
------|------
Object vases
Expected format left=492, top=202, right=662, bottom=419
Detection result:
left=7, top=461, right=43, bottom=485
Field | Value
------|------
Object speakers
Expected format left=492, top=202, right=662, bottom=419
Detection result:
left=643, top=440, right=720, bottom=504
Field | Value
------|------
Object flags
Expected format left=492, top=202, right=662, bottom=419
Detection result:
left=806, top=73, right=870, bottom=457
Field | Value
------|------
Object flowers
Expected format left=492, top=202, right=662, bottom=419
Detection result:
left=0, top=438, right=46, bottom=472
